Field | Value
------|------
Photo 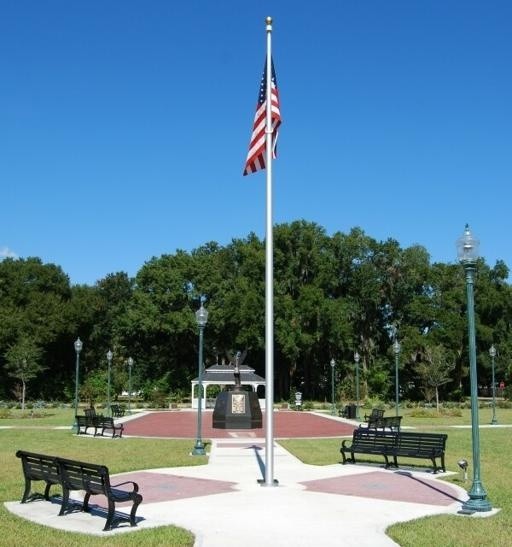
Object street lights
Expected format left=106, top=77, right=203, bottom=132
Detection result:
left=353, top=353, right=360, bottom=417
left=489, top=345, right=497, bottom=425
left=107, top=348, right=113, bottom=410
left=392, top=339, right=401, bottom=416
left=73, top=336, right=83, bottom=427
left=192, top=306, right=208, bottom=456
left=128, top=356, right=134, bottom=410
left=329, top=358, right=336, bottom=416
left=456, top=223, right=492, bottom=512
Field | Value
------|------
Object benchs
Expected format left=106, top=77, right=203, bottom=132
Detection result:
left=340, top=429, right=448, bottom=473
left=17, top=452, right=142, bottom=530
left=76, top=404, right=125, bottom=437
left=338, top=402, right=402, bottom=432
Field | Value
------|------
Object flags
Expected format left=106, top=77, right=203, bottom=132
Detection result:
left=243, top=53, right=281, bottom=176
